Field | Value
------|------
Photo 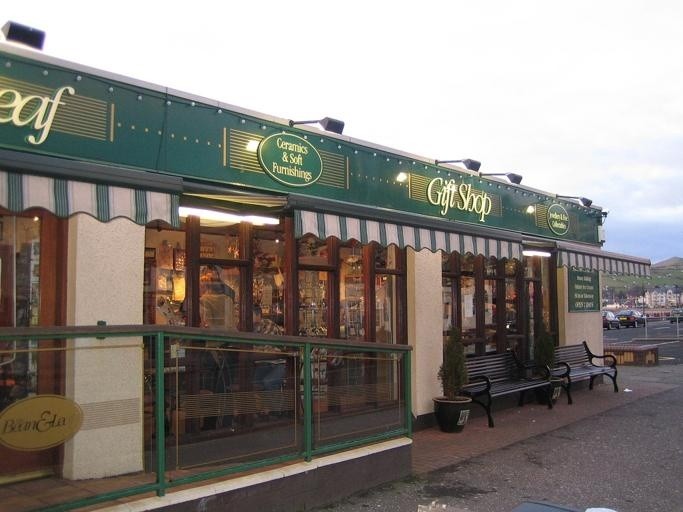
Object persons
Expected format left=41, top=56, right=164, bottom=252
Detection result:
left=179, top=265, right=288, bottom=414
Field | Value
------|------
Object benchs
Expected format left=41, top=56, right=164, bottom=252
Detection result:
left=455, top=353, right=554, bottom=428
left=552, top=341, right=618, bottom=404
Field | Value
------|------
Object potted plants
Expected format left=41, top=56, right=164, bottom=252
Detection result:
left=432, top=326, right=472, bottom=433
left=532, top=319, right=563, bottom=406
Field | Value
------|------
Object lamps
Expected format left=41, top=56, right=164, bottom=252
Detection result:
left=289, top=117, right=345, bottom=134
left=1, top=20, right=46, bottom=52
left=556, top=194, right=592, bottom=207
left=435, top=159, right=482, bottom=172
left=479, top=172, right=522, bottom=185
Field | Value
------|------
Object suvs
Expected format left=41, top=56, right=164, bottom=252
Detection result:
left=668, top=306, right=682, bottom=323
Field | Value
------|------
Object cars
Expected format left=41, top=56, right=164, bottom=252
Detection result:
left=493, top=316, right=533, bottom=350
left=615, top=310, right=647, bottom=329
left=602, top=311, right=621, bottom=330
